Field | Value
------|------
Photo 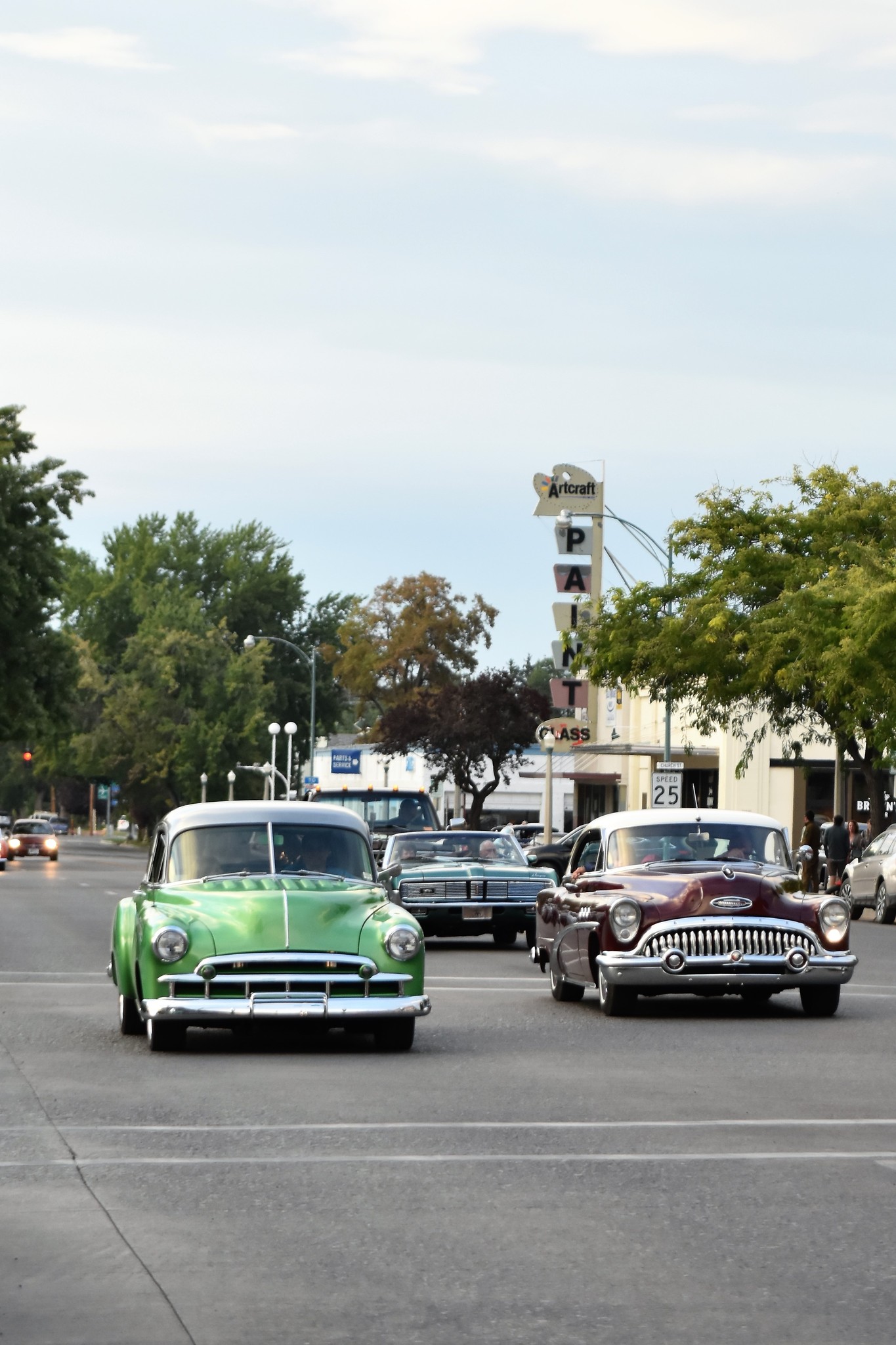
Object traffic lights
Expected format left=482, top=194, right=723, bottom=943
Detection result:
left=24, top=748, right=33, bottom=772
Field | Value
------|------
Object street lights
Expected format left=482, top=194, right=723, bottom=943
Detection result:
left=284, top=721, right=297, bottom=801
left=227, top=770, right=236, bottom=801
left=267, top=721, right=281, bottom=801
left=555, top=507, right=677, bottom=772
left=243, top=634, right=316, bottom=790
left=200, top=772, right=208, bottom=803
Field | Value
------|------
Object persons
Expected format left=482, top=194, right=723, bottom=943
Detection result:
left=799, top=810, right=821, bottom=893
left=394, top=798, right=423, bottom=826
left=861, top=818, right=872, bottom=849
left=398, top=841, right=417, bottom=859
left=823, top=814, right=850, bottom=895
left=521, top=820, right=527, bottom=825
left=847, top=819, right=862, bottom=863
left=480, top=840, right=497, bottom=859
left=571, top=840, right=634, bottom=879
left=507, top=822, right=513, bottom=825
left=715, top=836, right=753, bottom=859
left=282, top=834, right=356, bottom=879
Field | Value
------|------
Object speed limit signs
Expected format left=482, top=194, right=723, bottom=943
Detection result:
left=652, top=772, right=683, bottom=808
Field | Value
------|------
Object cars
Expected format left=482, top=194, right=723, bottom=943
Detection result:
left=0, top=828, right=9, bottom=871
left=375, top=829, right=559, bottom=952
left=4, top=818, right=58, bottom=861
left=0, top=810, right=14, bottom=832
left=105, top=799, right=430, bottom=1053
left=29, top=810, right=69, bottom=836
left=532, top=806, right=860, bottom=1017
left=839, top=823, right=896, bottom=924
left=794, top=821, right=871, bottom=892
